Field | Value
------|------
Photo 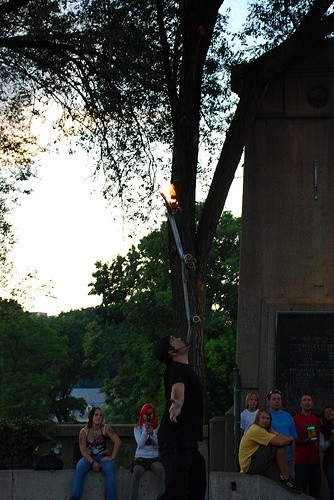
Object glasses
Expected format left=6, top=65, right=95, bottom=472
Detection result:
left=94, top=413, right=102, bottom=416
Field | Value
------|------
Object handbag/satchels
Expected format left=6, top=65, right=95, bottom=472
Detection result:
left=29, top=440, right=64, bottom=471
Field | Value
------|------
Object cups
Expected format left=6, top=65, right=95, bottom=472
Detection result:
left=307, top=426, right=315, bottom=439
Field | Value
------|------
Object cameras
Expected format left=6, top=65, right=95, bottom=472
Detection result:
left=147, top=413, right=152, bottom=422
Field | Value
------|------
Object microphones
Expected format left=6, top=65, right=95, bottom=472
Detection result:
left=173, top=343, right=189, bottom=353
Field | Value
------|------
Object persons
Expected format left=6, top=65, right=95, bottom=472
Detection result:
left=319, top=405, right=334, bottom=500
left=293, top=394, right=321, bottom=498
left=240, top=392, right=260, bottom=436
left=68, top=408, right=121, bottom=500
left=239, top=409, right=302, bottom=493
left=267, top=389, right=297, bottom=482
left=152, top=334, right=207, bottom=500
left=130, top=404, right=165, bottom=500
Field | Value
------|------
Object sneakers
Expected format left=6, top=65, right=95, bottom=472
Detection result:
left=278, top=475, right=302, bottom=494
